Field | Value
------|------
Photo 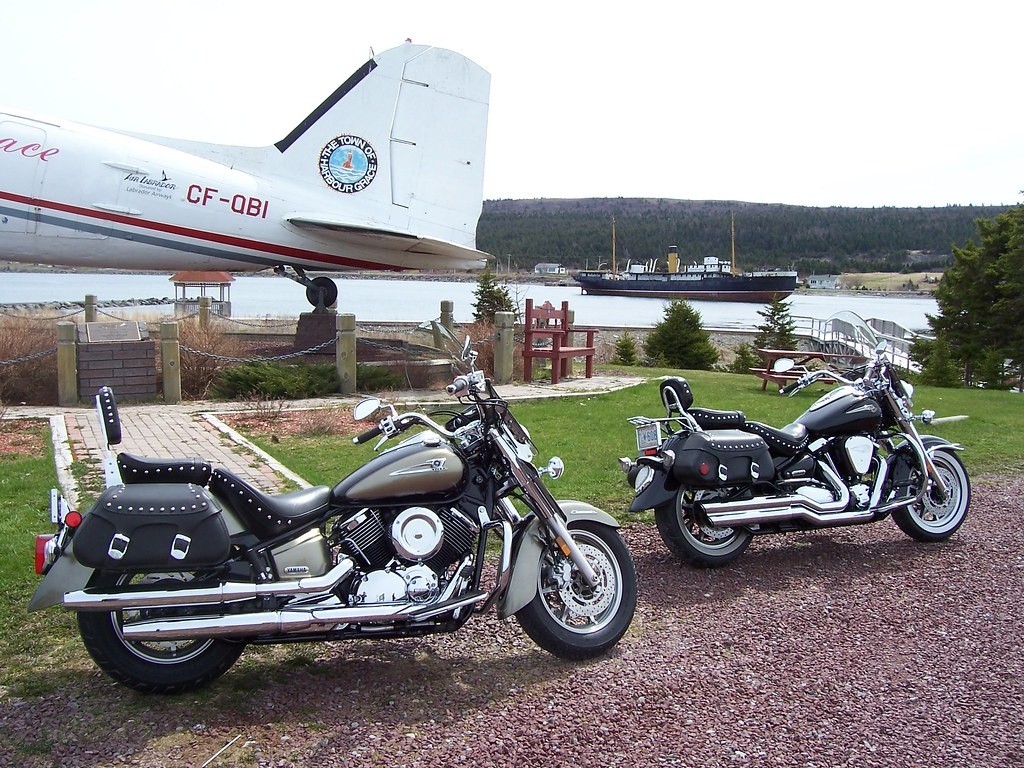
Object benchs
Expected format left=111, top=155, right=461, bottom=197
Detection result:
left=769, top=373, right=836, bottom=385
left=523, top=298, right=599, bottom=384
left=748, top=367, right=810, bottom=376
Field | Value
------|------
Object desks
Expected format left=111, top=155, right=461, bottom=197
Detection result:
left=757, top=349, right=828, bottom=392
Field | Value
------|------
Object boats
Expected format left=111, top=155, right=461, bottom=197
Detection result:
left=570, top=205, right=800, bottom=302
left=556, top=281, right=567, bottom=286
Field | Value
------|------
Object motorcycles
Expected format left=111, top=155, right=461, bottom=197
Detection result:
left=26, top=316, right=640, bottom=696
left=620, top=308, right=973, bottom=570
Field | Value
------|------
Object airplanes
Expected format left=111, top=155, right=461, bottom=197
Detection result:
left=0, top=41, right=496, bottom=276
